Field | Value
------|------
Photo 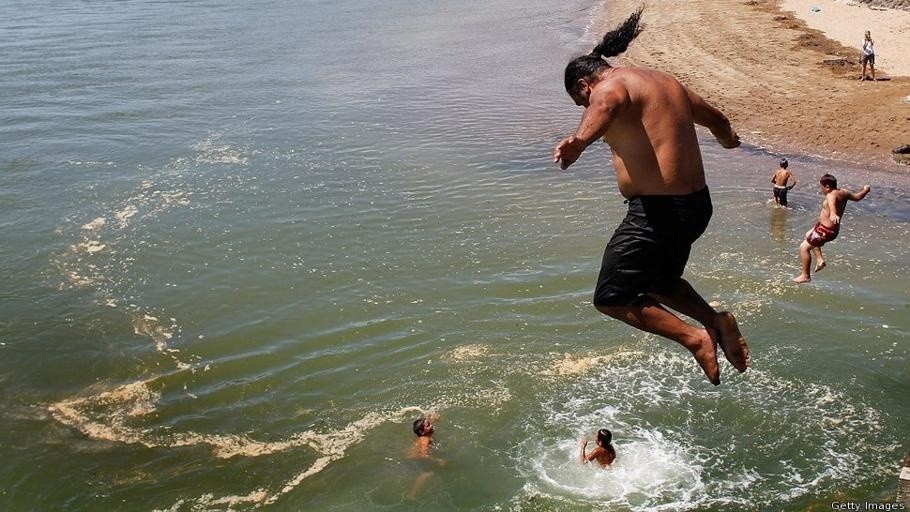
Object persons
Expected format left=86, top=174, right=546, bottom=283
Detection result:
left=581, top=429, right=616, bottom=468
left=553, top=1, right=749, bottom=387
left=404, top=418, right=447, bottom=500
left=770, top=157, right=797, bottom=206
left=861, top=30, right=877, bottom=83
left=792, top=173, right=872, bottom=282
